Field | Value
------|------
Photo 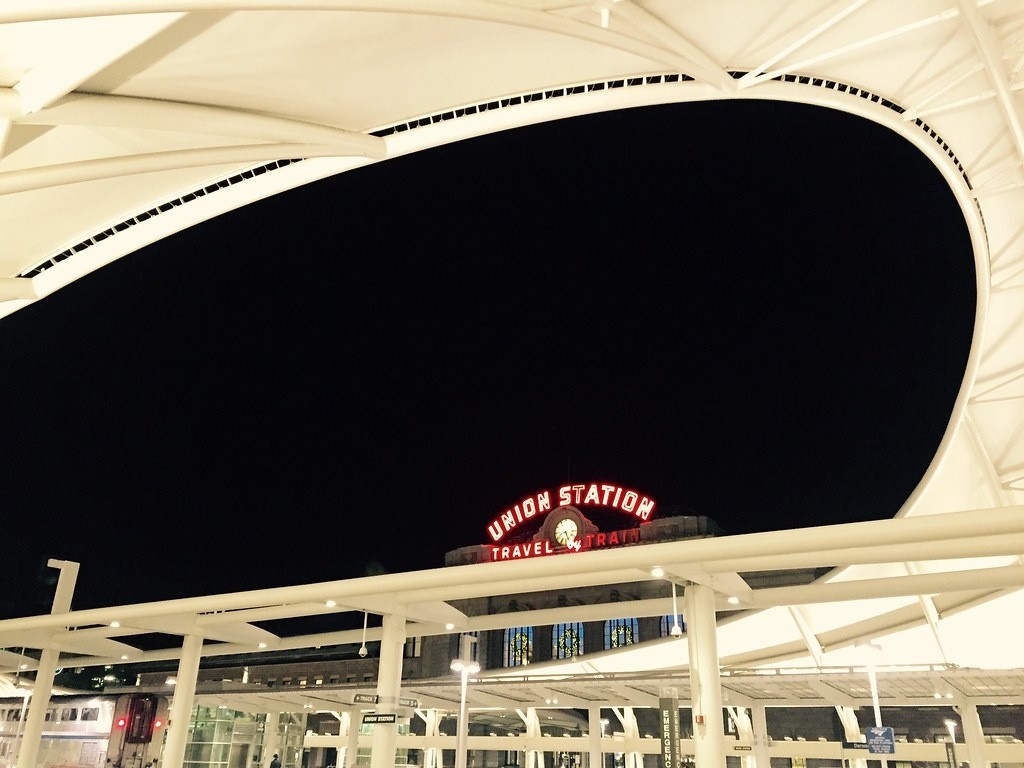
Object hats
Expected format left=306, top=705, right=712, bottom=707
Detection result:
left=273, top=754, right=278, bottom=757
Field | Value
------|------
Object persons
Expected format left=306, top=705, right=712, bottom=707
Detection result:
left=144, top=757, right=158, bottom=768
left=113, top=756, right=129, bottom=768
left=269, top=754, right=282, bottom=768
left=559, top=750, right=581, bottom=767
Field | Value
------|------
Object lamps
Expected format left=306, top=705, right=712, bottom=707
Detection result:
left=650, top=566, right=665, bottom=579
left=727, top=595, right=739, bottom=604
left=359, top=612, right=368, bottom=657
left=13, top=647, right=27, bottom=686
left=671, top=581, right=683, bottom=639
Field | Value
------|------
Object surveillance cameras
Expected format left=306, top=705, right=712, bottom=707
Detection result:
left=13, top=677, right=20, bottom=686
left=671, top=626, right=683, bottom=639
left=359, top=647, right=368, bottom=657
